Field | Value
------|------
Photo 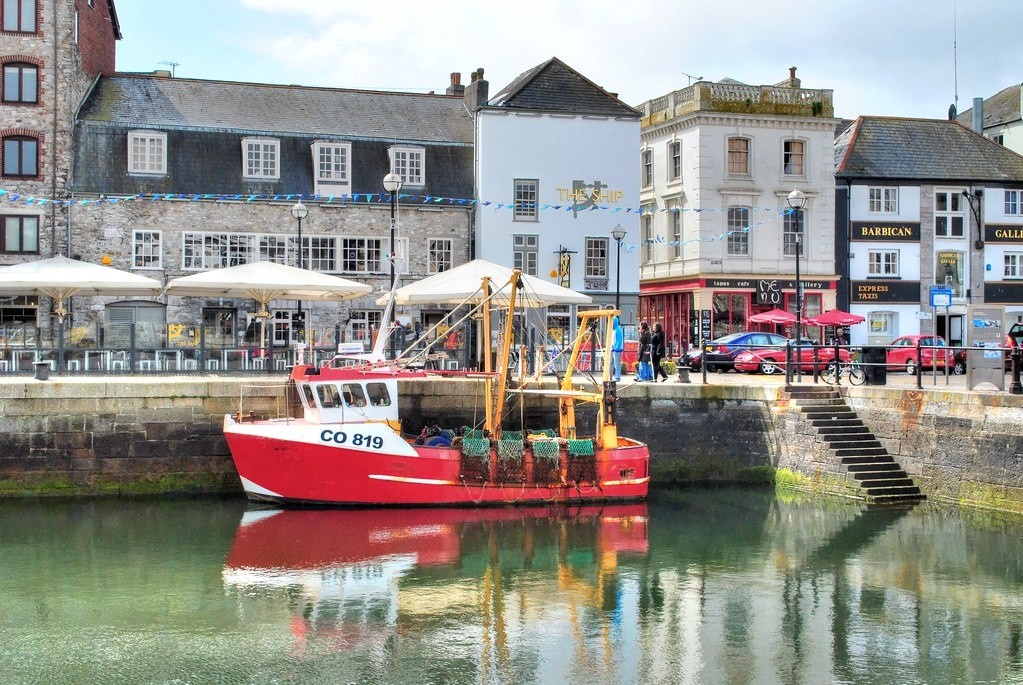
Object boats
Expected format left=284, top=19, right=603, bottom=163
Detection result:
left=221, top=501, right=650, bottom=680
left=221, top=268, right=652, bottom=507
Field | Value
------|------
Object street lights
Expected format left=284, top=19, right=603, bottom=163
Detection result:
left=290, top=199, right=309, bottom=339
left=611, top=223, right=626, bottom=323
left=786, top=186, right=806, bottom=381
left=382, top=169, right=402, bottom=357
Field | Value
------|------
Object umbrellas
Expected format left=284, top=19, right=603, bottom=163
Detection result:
left=0, top=253, right=162, bottom=372
left=379, top=258, right=594, bottom=373
left=164, top=259, right=371, bottom=366
left=807, top=309, right=865, bottom=336
left=747, top=308, right=798, bottom=334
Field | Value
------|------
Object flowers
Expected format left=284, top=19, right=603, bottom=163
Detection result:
left=620, top=361, right=676, bottom=375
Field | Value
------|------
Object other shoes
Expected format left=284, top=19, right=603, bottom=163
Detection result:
left=634, top=376, right=668, bottom=382
left=616, top=378, right=620, bottom=382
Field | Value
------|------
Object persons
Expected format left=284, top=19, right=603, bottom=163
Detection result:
left=246, top=319, right=267, bottom=360
left=834, top=328, right=850, bottom=350
left=634, top=322, right=653, bottom=382
left=610, top=316, right=623, bottom=382
left=394, top=320, right=426, bottom=348
left=650, top=322, right=668, bottom=382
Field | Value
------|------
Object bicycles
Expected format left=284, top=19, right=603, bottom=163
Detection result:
left=819, top=358, right=866, bottom=386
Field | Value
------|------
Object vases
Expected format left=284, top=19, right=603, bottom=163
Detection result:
left=621, top=367, right=628, bottom=375
left=662, top=362, right=669, bottom=373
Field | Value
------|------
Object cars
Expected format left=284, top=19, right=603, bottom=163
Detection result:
left=678, top=348, right=704, bottom=371
left=954, top=346, right=1012, bottom=376
left=734, top=337, right=851, bottom=375
left=705, top=332, right=790, bottom=374
left=885, top=335, right=955, bottom=375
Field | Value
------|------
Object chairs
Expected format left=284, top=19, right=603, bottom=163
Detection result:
left=508, top=350, right=605, bottom=373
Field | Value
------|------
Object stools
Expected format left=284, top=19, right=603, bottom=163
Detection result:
left=113, top=361, right=124, bottom=371
left=319, top=360, right=330, bottom=368
left=249, top=358, right=263, bottom=370
left=447, top=361, right=458, bottom=370
left=275, top=359, right=287, bottom=370
left=68, top=359, right=80, bottom=371
left=140, top=360, right=150, bottom=370
left=208, top=359, right=218, bottom=370
left=43, top=360, right=56, bottom=371
left=185, top=359, right=198, bottom=370
left=0, top=360, right=11, bottom=372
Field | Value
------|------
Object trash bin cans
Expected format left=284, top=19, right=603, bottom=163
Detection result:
left=862, top=346, right=886, bottom=385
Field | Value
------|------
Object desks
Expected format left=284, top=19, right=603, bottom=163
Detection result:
left=12, top=350, right=40, bottom=372
left=156, top=350, right=184, bottom=371
left=85, top=350, right=114, bottom=371
left=221, top=349, right=249, bottom=371
